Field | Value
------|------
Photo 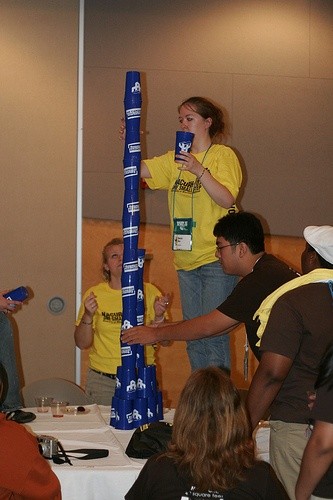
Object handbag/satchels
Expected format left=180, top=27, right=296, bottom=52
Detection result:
left=126, top=421, right=173, bottom=459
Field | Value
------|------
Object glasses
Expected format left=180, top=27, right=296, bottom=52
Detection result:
left=216, top=243, right=239, bottom=252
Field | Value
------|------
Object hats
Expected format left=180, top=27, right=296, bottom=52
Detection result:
left=304, top=225, right=333, bottom=264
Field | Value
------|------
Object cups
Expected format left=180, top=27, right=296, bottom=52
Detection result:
left=34, top=397, right=54, bottom=413
left=109, top=70, right=166, bottom=431
left=174, top=131, right=195, bottom=161
left=50, top=401, right=68, bottom=417
left=3, top=286, right=29, bottom=308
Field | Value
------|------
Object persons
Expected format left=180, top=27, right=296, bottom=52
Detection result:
left=0, top=363, right=62, bottom=500
left=0, top=290, right=36, bottom=423
left=125, top=365, right=291, bottom=500
left=74, top=238, right=173, bottom=406
left=121, top=210, right=333, bottom=500
left=119, top=95, right=243, bottom=372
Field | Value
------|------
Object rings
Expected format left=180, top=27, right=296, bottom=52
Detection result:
left=165, top=301, right=169, bottom=304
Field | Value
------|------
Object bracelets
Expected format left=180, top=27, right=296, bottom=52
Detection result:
left=81, top=318, right=93, bottom=325
left=153, top=316, right=164, bottom=324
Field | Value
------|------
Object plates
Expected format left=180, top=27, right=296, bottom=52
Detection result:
left=64, top=403, right=99, bottom=416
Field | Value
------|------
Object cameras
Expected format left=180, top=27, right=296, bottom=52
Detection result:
left=36, top=434, right=58, bottom=459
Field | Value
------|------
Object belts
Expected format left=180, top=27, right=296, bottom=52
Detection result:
left=91, top=369, right=117, bottom=380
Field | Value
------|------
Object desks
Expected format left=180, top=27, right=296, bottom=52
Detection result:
left=13, top=404, right=269, bottom=500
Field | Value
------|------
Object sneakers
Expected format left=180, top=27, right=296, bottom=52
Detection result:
left=2, top=409, right=36, bottom=423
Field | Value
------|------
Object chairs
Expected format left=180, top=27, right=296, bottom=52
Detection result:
left=21, top=378, right=85, bottom=407
